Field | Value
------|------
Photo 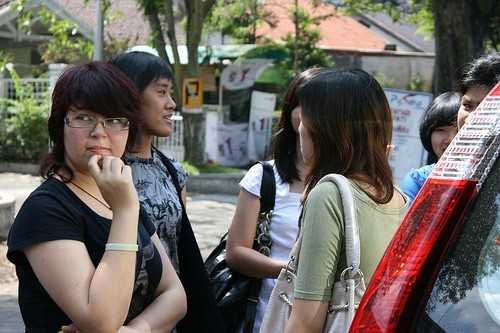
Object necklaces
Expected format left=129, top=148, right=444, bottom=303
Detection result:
left=57, top=172, right=112, bottom=211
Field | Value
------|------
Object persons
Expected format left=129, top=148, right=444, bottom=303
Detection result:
left=108, top=51, right=226, bottom=333
left=457, top=52, right=500, bottom=131
left=402, top=92, right=462, bottom=210
left=225, top=68, right=394, bottom=333
left=283, top=68, right=410, bottom=333
left=7, top=60, right=187, bottom=333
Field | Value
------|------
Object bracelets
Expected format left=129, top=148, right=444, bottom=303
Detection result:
left=105, top=243, right=138, bottom=251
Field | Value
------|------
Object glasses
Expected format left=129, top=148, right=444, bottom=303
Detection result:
left=63, top=115, right=131, bottom=131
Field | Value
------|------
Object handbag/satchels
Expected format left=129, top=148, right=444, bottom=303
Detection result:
left=151, top=143, right=225, bottom=333
left=205, top=161, right=276, bottom=333
left=259, top=173, right=366, bottom=333
